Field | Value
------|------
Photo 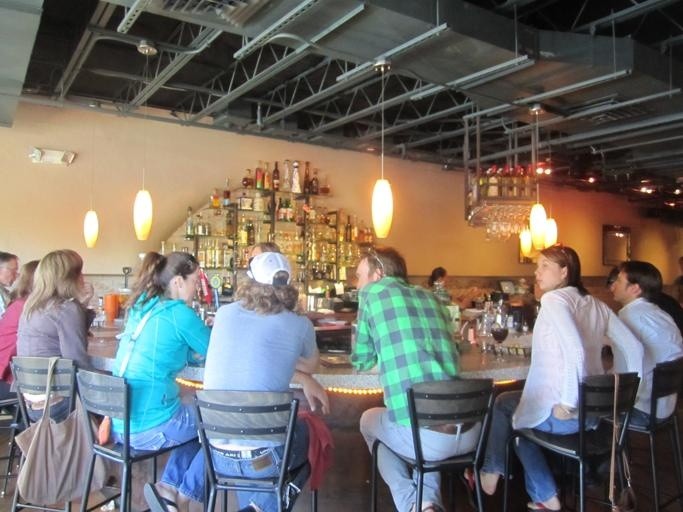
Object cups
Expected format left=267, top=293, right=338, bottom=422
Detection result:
left=103, top=293, right=118, bottom=323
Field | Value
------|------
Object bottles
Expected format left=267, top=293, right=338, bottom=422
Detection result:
left=156, top=158, right=373, bottom=281
left=476, top=166, right=535, bottom=199
left=98, top=297, right=103, bottom=309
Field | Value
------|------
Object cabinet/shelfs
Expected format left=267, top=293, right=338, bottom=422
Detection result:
left=172, top=183, right=376, bottom=302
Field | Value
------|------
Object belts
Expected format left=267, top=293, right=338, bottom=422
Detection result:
left=425, top=418, right=476, bottom=434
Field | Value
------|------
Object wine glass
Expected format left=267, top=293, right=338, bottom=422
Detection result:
left=476, top=296, right=528, bottom=355
left=481, top=203, right=533, bottom=246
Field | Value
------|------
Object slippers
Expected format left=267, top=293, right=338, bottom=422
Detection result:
left=144, top=483, right=178, bottom=512
left=527, top=500, right=553, bottom=512
left=463, top=465, right=492, bottom=509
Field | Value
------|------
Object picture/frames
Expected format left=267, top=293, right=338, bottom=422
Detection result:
left=600, top=223, right=631, bottom=267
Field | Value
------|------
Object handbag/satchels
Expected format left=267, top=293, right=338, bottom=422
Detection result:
left=14, top=400, right=110, bottom=504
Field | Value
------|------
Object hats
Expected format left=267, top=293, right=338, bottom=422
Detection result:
left=247, top=251, right=292, bottom=286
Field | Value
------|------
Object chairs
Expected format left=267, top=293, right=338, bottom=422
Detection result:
left=11, top=353, right=125, bottom=510
left=594, top=354, right=683, bottom=510
left=190, top=386, right=322, bottom=511
left=74, top=366, right=208, bottom=512
left=2, top=376, right=28, bottom=499
left=498, top=372, right=648, bottom=511
left=362, top=375, right=500, bottom=510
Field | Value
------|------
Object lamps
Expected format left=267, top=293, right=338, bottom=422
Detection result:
left=131, top=38, right=156, bottom=243
left=82, top=102, right=100, bottom=249
left=528, top=100, right=548, bottom=252
left=517, top=218, right=559, bottom=267
left=370, top=54, right=395, bottom=241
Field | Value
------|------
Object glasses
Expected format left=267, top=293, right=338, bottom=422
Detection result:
left=552, top=242, right=568, bottom=260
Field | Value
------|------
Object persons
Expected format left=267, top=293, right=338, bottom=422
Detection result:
left=348, top=246, right=482, bottom=512
left=111, top=252, right=208, bottom=511
left=202, top=250, right=330, bottom=512
left=428, top=267, right=447, bottom=287
left=204, top=242, right=280, bottom=327
left=457, top=244, right=645, bottom=512
left=1, top=250, right=112, bottom=444
left=548, top=257, right=683, bottom=511
